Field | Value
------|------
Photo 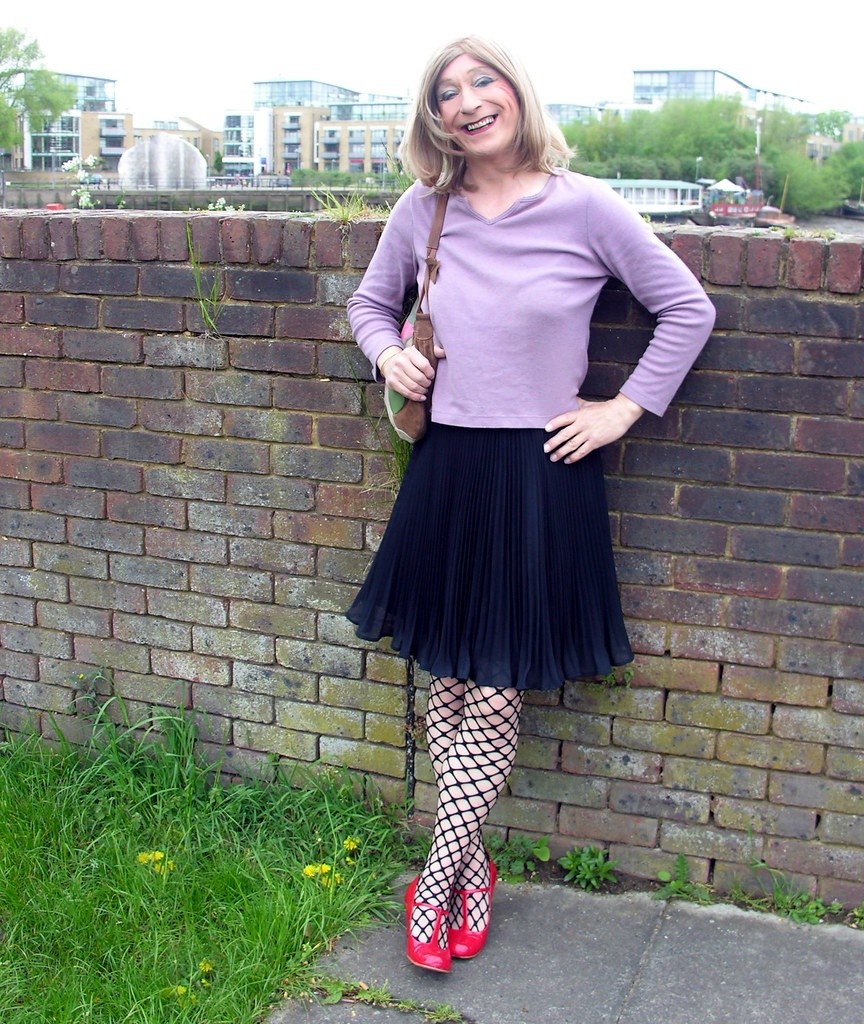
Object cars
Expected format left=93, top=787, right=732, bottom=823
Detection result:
left=80, top=174, right=102, bottom=184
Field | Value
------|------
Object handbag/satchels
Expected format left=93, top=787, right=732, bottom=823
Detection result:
left=384, top=184, right=452, bottom=444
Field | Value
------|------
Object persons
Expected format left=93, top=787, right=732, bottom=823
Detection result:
left=346, top=36, right=715, bottom=968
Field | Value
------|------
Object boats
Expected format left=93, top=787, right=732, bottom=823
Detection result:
left=843, top=177, right=864, bottom=214
left=689, top=119, right=797, bottom=226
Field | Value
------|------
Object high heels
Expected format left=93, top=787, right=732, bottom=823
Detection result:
left=405, top=874, right=452, bottom=974
left=447, top=846, right=496, bottom=959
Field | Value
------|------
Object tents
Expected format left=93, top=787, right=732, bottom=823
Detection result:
left=707, top=178, right=743, bottom=203
